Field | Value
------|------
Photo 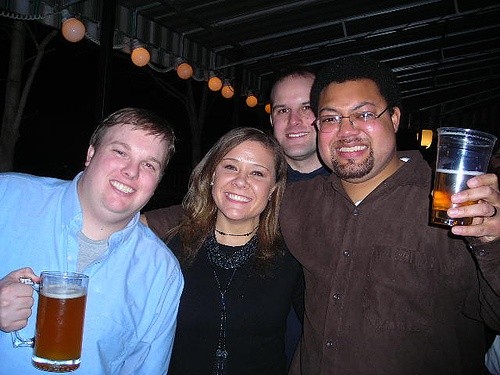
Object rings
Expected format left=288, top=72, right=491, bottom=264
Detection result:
left=490, top=206, right=497, bottom=217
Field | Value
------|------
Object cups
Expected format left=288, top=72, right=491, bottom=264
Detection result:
left=427, top=126, right=498, bottom=229
left=10, top=271, right=90, bottom=373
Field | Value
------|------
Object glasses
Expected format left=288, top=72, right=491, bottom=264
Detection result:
left=311, top=106, right=391, bottom=134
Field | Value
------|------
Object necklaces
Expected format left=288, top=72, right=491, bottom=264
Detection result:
left=203, top=240, right=257, bottom=374
left=212, top=227, right=258, bottom=237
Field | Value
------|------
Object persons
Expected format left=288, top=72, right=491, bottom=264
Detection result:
left=141, top=56, right=500, bottom=375
left=0, top=106, right=184, bottom=375
